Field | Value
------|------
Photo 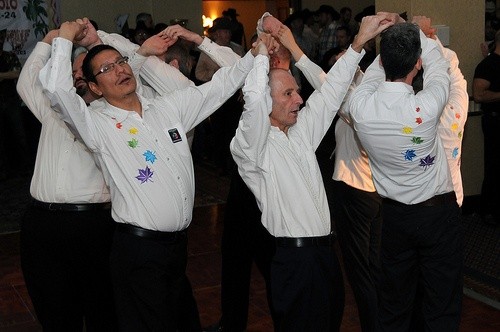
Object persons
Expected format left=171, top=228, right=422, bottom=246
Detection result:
left=0, top=44, right=21, bottom=150
left=472, top=30, right=500, bottom=222
left=72, top=3, right=469, bottom=332
left=80, top=24, right=194, bottom=150
left=348, top=12, right=462, bottom=332
left=230, top=15, right=393, bottom=332
left=272, top=22, right=388, bottom=332
left=16, top=29, right=178, bottom=332
left=40, top=21, right=279, bottom=332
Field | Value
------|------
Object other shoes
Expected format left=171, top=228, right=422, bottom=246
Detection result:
left=202, top=323, right=227, bottom=332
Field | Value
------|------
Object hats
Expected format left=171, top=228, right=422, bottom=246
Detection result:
left=223, top=8, right=240, bottom=16
left=212, top=17, right=236, bottom=31
left=115, top=14, right=128, bottom=33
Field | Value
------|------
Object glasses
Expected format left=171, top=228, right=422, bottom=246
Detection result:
left=94, top=56, right=129, bottom=77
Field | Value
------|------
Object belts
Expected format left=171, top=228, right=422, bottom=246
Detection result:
left=382, top=191, right=457, bottom=207
left=46, top=203, right=109, bottom=212
left=129, top=226, right=186, bottom=242
left=293, top=232, right=337, bottom=247
